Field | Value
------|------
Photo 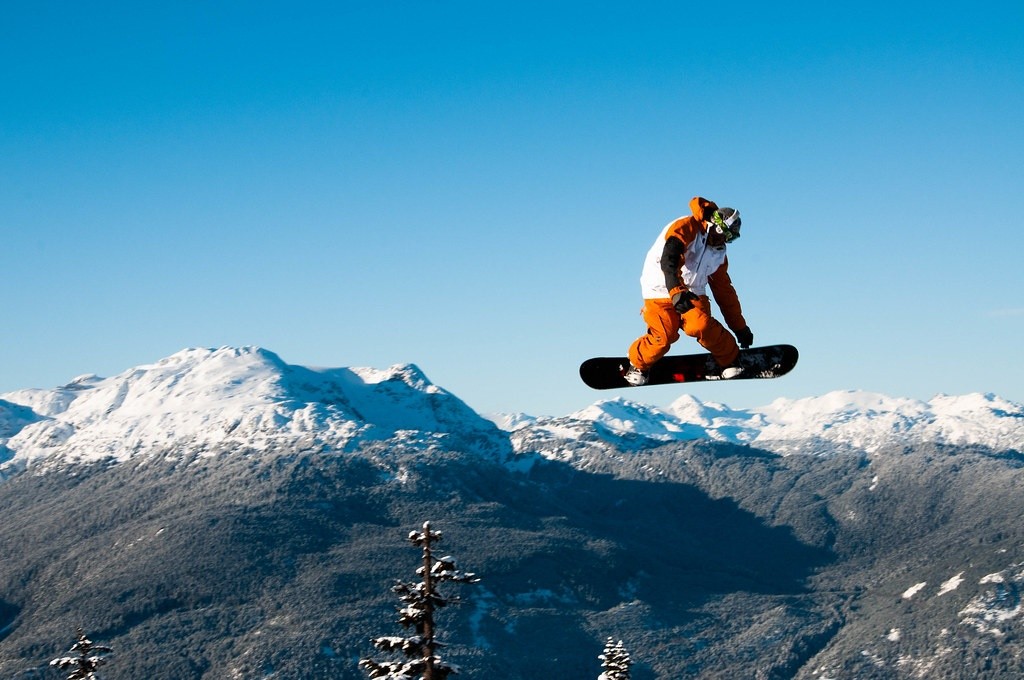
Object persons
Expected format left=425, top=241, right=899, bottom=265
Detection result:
left=621, top=198, right=754, bottom=385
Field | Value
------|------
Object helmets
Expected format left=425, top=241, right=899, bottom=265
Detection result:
left=710, top=208, right=741, bottom=243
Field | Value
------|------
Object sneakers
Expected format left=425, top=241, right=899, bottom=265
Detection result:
left=720, top=356, right=744, bottom=379
left=624, top=361, right=650, bottom=386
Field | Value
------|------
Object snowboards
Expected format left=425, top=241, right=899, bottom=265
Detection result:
left=581, top=344, right=801, bottom=390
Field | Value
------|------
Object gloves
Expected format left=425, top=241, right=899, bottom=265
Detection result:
left=734, top=325, right=754, bottom=349
left=672, top=291, right=699, bottom=314
